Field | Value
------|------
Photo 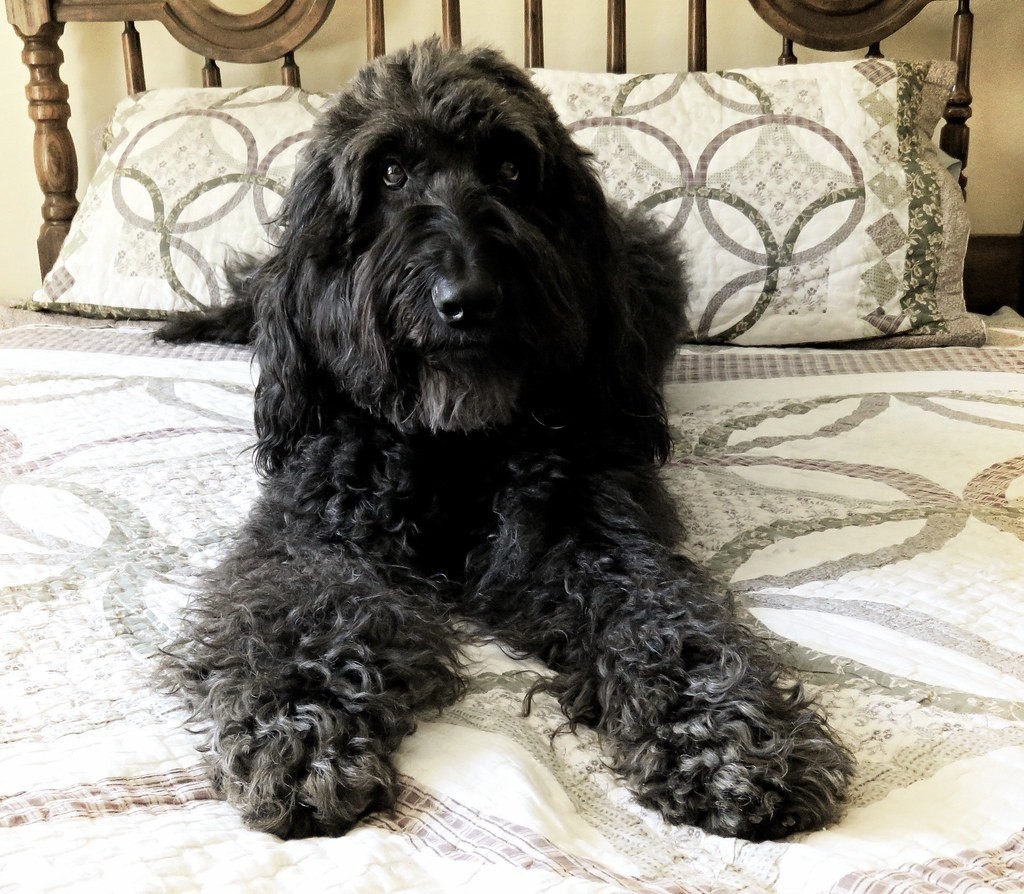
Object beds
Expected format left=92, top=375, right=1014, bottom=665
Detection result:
left=0, top=0, right=1024, bottom=894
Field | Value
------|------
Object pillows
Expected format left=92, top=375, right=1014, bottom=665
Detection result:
left=28, top=81, right=334, bottom=317
left=520, top=57, right=986, bottom=351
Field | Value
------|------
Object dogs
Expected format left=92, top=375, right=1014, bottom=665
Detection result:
left=146, top=34, right=862, bottom=845
